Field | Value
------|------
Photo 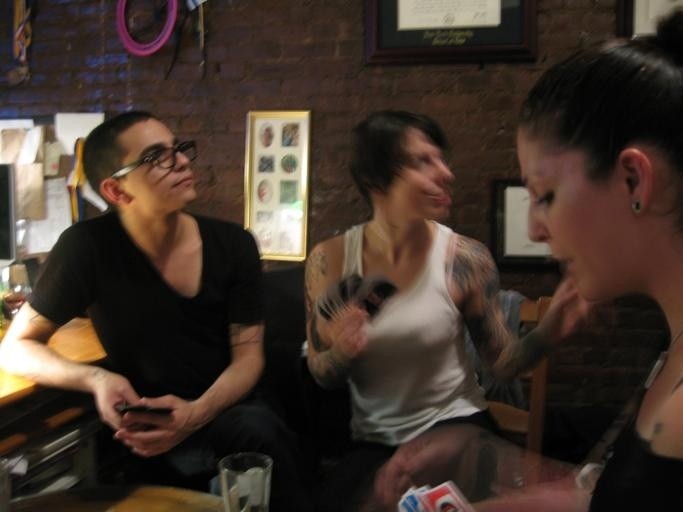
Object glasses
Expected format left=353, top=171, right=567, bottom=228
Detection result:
left=109, top=139, right=198, bottom=181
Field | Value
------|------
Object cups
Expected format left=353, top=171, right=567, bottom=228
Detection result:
left=218, top=452, right=274, bottom=512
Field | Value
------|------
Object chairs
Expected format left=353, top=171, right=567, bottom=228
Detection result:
left=479, top=295, right=556, bottom=454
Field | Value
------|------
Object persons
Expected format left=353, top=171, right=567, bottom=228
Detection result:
left=371, top=32, right=683, bottom=510
left=305, top=107, right=589, bottom=511
left=2, top=111, right=306, bottom=510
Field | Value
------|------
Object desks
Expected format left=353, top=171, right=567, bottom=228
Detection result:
left=0, top=317, right=112, bottom=512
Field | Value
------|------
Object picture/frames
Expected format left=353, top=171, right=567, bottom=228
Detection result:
left=364, top=0, right=539, bottom=64
left=614, top=0, right=683, bottom=45
left=491, top=175, right=561, bottom=272
left=243, top=109, right=313, bottom=262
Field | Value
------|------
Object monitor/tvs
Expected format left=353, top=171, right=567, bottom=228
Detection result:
left=1, top=162, right=18, bottom=270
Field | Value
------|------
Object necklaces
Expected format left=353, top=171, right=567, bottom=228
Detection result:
left=643, top=329, right=682, bottom=390
